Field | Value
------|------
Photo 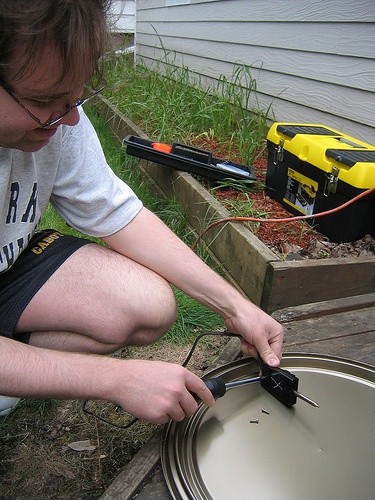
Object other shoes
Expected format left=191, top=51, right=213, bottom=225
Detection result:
left=0, top=395, right=21, bottom=416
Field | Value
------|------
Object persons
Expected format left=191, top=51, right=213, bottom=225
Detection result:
left=0, top=0, right=285, bottom=424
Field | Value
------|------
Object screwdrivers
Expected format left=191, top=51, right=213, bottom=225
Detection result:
left=191, top=375, right=267, bottom=405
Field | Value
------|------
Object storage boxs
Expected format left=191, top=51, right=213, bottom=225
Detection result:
left=264, top=123, right=375, bottom=241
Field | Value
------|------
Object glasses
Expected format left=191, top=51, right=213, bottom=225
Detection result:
left=0, top=65, right=107, bottom=129
